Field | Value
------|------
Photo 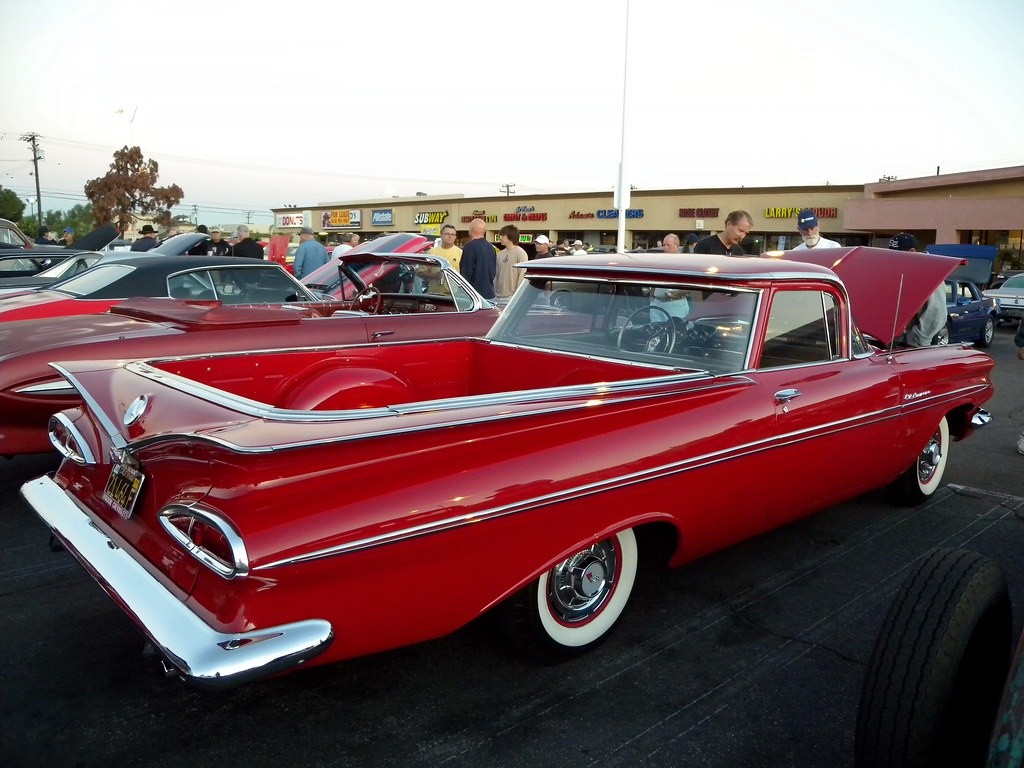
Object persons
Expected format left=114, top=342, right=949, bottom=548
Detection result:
left=130, top=224, right=158, bottom=252
left=1014, top=315, right=1024, bottom=454
left=889, top=233, right=947, bottom=349
left=188, top=225, right=587, bottom=311
left=57, top=227, right=76, bottom=246
left=649, top=211, right=753, bottom=323
left=35, top=226, right=57, bottom=244
left=792, top=210, right=842, bottom=250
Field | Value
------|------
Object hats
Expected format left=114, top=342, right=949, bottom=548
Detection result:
left=888, top=232, right=920, bottom=251
left=688, top=233, right=698, bottom=242
left=533, top=235, right=549, bottom=245
left=296, top=227, right=314, bottom=235
left=64, top=227, right=74, bottom=232
left=798, top=209, right=817, bottom=230
left=138, top=225, right=158, bottom=233
left=210, top=225, right=221, bottom=232
left=571, top=240, right=583, bottom=245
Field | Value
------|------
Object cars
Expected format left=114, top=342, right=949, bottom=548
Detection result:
left=20, top=239, right=986, bottom=692
left=924, top=242, right=997, bottom=349
left=0, top=218, right=634, bottom=455
left=980, top=272, right=1024, bottom=319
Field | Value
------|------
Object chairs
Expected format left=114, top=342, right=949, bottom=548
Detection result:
left=198, top=289, right=223, bottom=302
left=170, top=287, right=193, bottom=300
